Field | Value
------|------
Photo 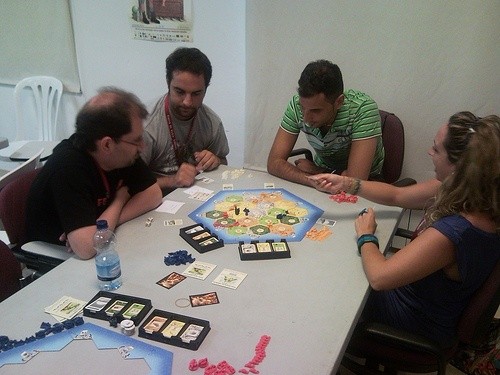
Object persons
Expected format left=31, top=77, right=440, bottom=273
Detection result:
left=267, top=60, right=384, bottom=188
left=24, top=86, right=162, bottom=259
left=141, top=47, right=230, bottom=188
left=139, top=0, right=160, bottom=24
left=307, top=111, right=500, bottom=351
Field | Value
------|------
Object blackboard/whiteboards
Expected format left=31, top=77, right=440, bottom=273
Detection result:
left=0, top=0, right=83, bottom=94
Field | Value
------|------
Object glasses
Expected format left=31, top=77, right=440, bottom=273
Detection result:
left=119, top=132, right=149, bottom=150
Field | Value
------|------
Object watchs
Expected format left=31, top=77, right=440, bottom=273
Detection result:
left=358, top=237, right=379, bottom=254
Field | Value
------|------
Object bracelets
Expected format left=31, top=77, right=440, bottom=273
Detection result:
left=344, top=175, right=360, bottom=195
left=357, top=234, right=378, bottom=245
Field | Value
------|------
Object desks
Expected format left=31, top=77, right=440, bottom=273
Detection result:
left=0, top=165, right=406, bottom=375
left=10, top=140, right=60, bottom=161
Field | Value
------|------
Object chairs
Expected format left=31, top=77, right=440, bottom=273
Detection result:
left=288, top=110, right=417, bottom=187
left=0, top=76, right=64, bottom=170
left=0, top=165, right=74, bottom=303
left=340, top=226, right=500, bottom=375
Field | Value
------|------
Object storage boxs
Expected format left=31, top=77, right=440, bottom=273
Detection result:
left=180, top=223, right=225, bottom=253
left=84, top=290, right=152, bottom=326
left=138, top=309, right=212, bottom=351
left=239, top=239, right=292, bottom=261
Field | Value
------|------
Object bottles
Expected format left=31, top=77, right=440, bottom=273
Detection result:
left=93, top=219, right=123, bottom=292
